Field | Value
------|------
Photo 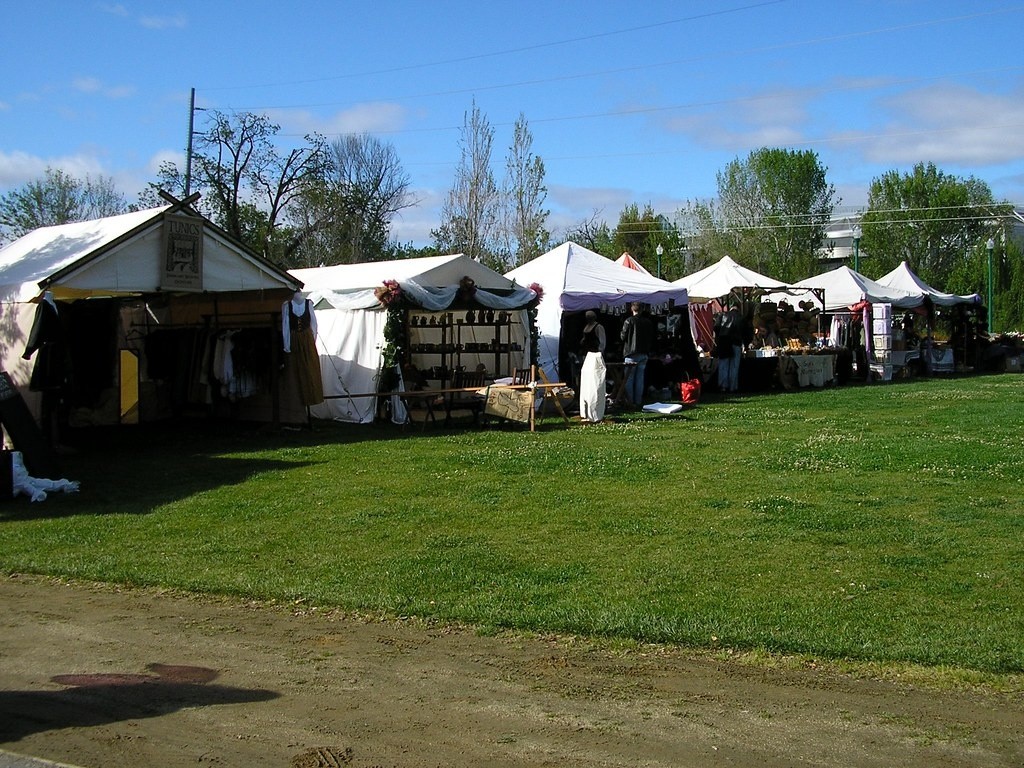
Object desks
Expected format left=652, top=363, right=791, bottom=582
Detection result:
left=737, top=354, right=838, bottom=394
left=891, top=349, right=920, bottom=382
left=921, top=349, right=955, bottom=373
left=605, top=362, right=638, bottom=415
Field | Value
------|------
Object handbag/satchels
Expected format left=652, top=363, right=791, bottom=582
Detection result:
left=681, top=379, right=701, bottom=404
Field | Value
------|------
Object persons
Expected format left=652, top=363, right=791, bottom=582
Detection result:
left=282, top=293, right=324, bottom=405
left=620, top=303, right=653, bottom=405
left=581, top=312, right=606, bottom=359
left=712, top=304, right=746, bottom=393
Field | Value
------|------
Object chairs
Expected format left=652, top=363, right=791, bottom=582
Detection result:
left=443, top=369, right=488, bottom=430
left=492, top=367, right=532, bottom=429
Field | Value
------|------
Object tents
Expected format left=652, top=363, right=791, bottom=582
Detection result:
left=1, top=191, right=306, bottom=443
left=794, top=266, right=927, bottom=358
left=286, top=255, right=543, bottom=422
left=875, top=260, right=981, bottom=365
left=502, top=241, right=690, bottom=407
left=672, top=257, right=822, bottom=341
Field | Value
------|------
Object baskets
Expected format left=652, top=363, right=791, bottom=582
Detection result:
left=754, top=301, right=822, bottom=342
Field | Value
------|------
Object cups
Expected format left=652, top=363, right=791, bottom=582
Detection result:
left=457, top=319, right=463, bottom=324
left=411, top=338, right=522, bottom=350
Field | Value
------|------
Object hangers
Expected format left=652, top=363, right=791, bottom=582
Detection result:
left=126, top=322, right=145, bottom=340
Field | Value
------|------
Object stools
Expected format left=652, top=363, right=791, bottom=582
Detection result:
left=400, top=394, right=439, bottom=433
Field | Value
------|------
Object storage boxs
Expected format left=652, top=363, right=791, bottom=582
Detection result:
left=869, top=302, right=906, bottom=382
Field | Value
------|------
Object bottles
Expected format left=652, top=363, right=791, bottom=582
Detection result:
left=411, top=363, right=467, bottom=380
left=408, top=307, right=507, bottom=325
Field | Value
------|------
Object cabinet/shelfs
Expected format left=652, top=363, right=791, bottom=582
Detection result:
left=403, top=313, right=524, bottom=401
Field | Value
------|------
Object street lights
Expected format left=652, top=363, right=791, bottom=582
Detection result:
left=853, top=226, right=862, bottom=273
left=987, top=239, right=994, bottom=332
left=656, top=243, right=663, bottom=279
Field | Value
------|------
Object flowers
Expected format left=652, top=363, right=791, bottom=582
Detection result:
left=374, top=279, right=405, bottom=392
left=528, top=281, right=546, bottom=382
